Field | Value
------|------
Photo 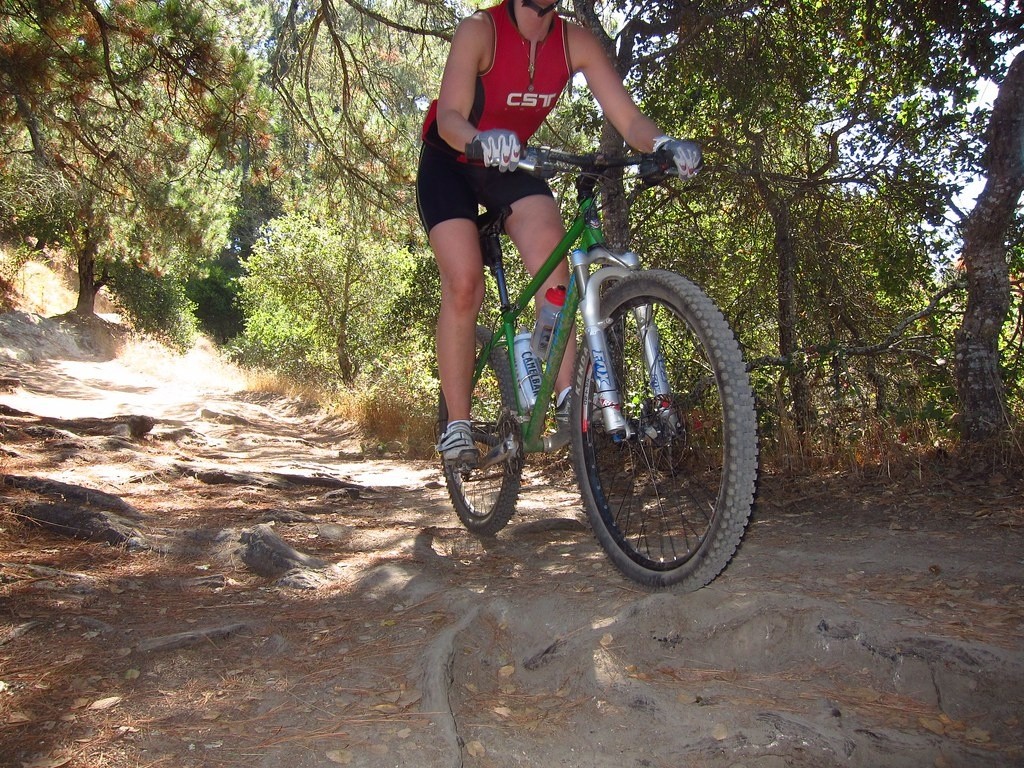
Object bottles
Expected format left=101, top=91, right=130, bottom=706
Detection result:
left=511, top=324, right=541, bottom=409
left=529, top=284, right=566, bottom=358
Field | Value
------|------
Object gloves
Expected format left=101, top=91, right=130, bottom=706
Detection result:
left=472, top=128, right=522, bottom=173
left=652, top=135, right=705, bottom=181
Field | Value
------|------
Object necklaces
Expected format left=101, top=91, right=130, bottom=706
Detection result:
left=513, top=8, right=555, bottom=73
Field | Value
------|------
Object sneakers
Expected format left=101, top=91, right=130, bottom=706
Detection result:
left=436, top=421, right=481, bottom=463
left=553, top=389, right=624, bottom=431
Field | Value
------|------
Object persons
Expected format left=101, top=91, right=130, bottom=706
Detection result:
left=414, top=1, right=704, bottom=465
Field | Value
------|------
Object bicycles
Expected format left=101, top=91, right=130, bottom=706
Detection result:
left=434, top=142, right=761, bottom=594
